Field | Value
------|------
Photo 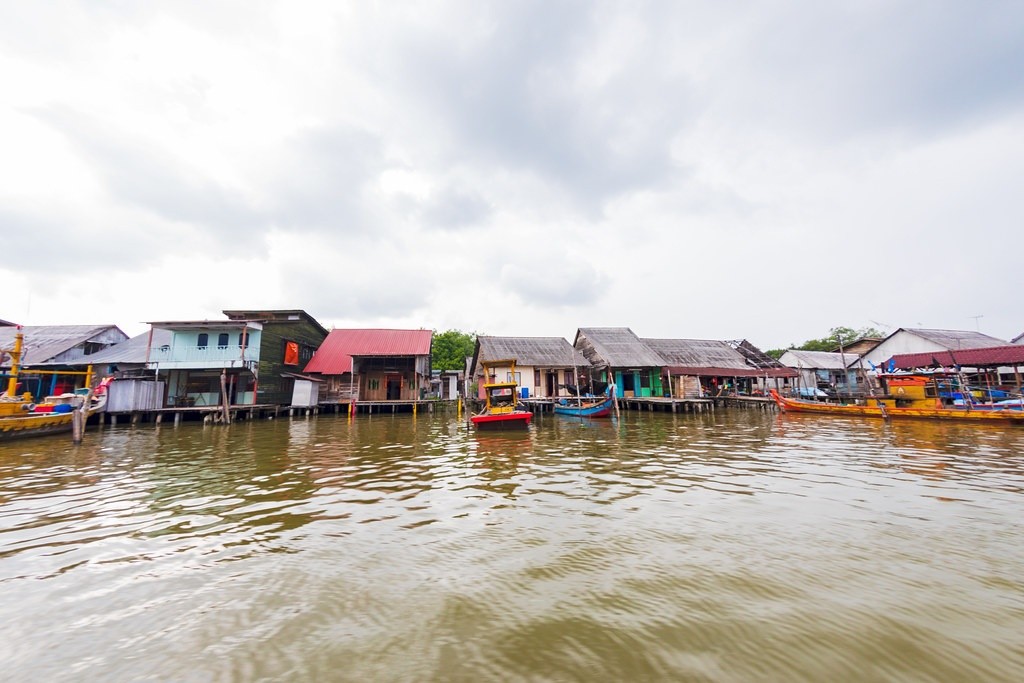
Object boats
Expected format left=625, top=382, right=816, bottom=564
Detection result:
left=552, top=395, right=614, bottom=418
left=768, top=385, right=1024, bottom=424
left=0, top=384, right=107, bottom=439
left=470, top=360, right=534, bottom=431
